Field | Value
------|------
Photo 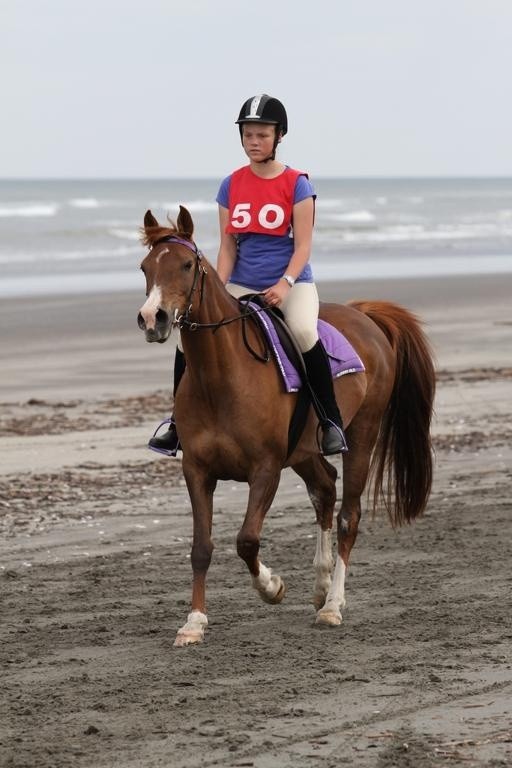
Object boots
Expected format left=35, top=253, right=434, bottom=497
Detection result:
left=149, top=345, right=182, bottom=449
left=301, top=339, right=344, bottom=453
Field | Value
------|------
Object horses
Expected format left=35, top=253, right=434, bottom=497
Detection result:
left=136, top=204, right=436, bottom=648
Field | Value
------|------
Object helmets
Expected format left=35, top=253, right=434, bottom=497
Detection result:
left=235, top=94, right=287, bottom=136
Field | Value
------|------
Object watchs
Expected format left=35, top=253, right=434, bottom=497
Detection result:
left=281, top=275, right=295, bottom=288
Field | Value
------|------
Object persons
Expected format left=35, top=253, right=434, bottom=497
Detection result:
left=148, top=95, right=345, bottom=455
